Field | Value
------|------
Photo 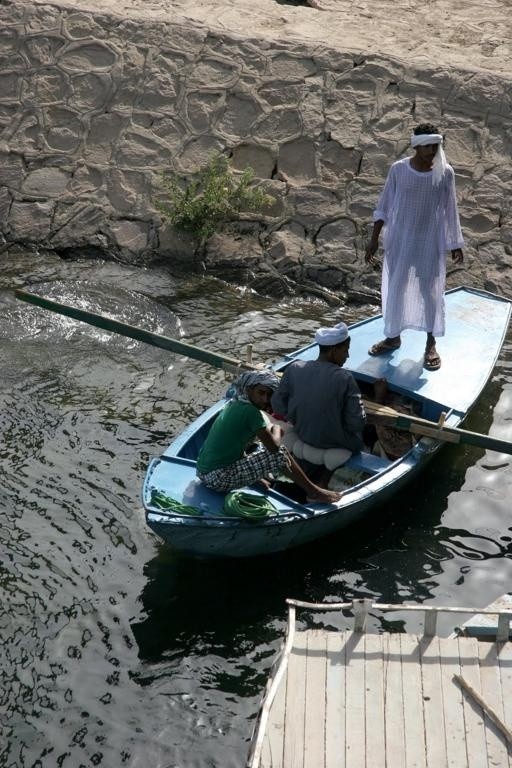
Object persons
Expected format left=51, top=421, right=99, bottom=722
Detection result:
left=196, top=368, right=342, bottom=504
left=270, top=321, right=368, bottom=452
left=364, top=123, right=464, bottom=369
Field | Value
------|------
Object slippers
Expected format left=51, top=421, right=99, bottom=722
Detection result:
left=368, top=341, right=400, bottom=355
left=425, top=351, right=440, bottom=370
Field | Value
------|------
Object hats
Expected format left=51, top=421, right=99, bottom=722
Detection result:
left=376, top=404, right=417, bottom=457
left=316, top=321, right=349, bottom=345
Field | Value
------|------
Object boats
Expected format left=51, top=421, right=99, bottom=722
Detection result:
left=142, top=286, right=512, bottom=559
